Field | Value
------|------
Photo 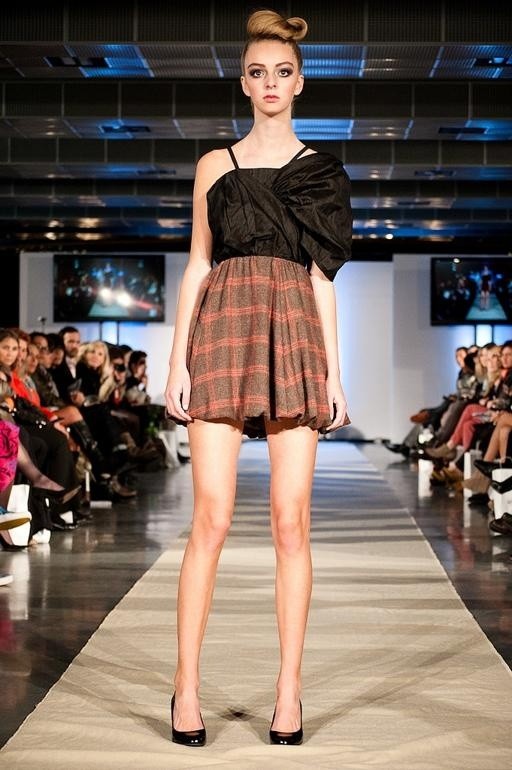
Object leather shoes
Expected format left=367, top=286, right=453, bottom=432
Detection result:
left=490, top=476, right=512, bottom=494
left=97, top=440, right=170, bottom=498
left=474, top=459, right=512, bottom=479
left=490, top=512, right=512, bottom=534
left=469, top=492, right=490, bottom=505
left=177, top=452, right=190, bottom=461
left=462, top=471, right=490, bottom=493
left=270, top=698, right=303, bottom=745
left=385, top=441, right=464, bottom=492
left=171, top=691, right=206, bottom=746
left=51, top=518, right=79, bottom=531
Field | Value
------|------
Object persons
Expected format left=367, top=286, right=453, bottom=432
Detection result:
left=0, top=321, right=172, bottom=550
left=375, top=336, right=511, bottom=541
left=435, top=265, right=510, bottom=324
left=54, top=258, right=162, bottom=321
left=162, top=7, right=355, bottom=747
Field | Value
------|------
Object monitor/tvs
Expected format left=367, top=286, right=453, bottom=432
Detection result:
left=52, top=254, right=165, bottom=323
left=430, top=257, right=511, bottom=327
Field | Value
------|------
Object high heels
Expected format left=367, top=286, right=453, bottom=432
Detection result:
left=31, top=484, right=82, bottom=513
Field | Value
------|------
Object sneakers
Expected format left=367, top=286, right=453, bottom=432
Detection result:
left=1, top=511, right=31, bottom=530
